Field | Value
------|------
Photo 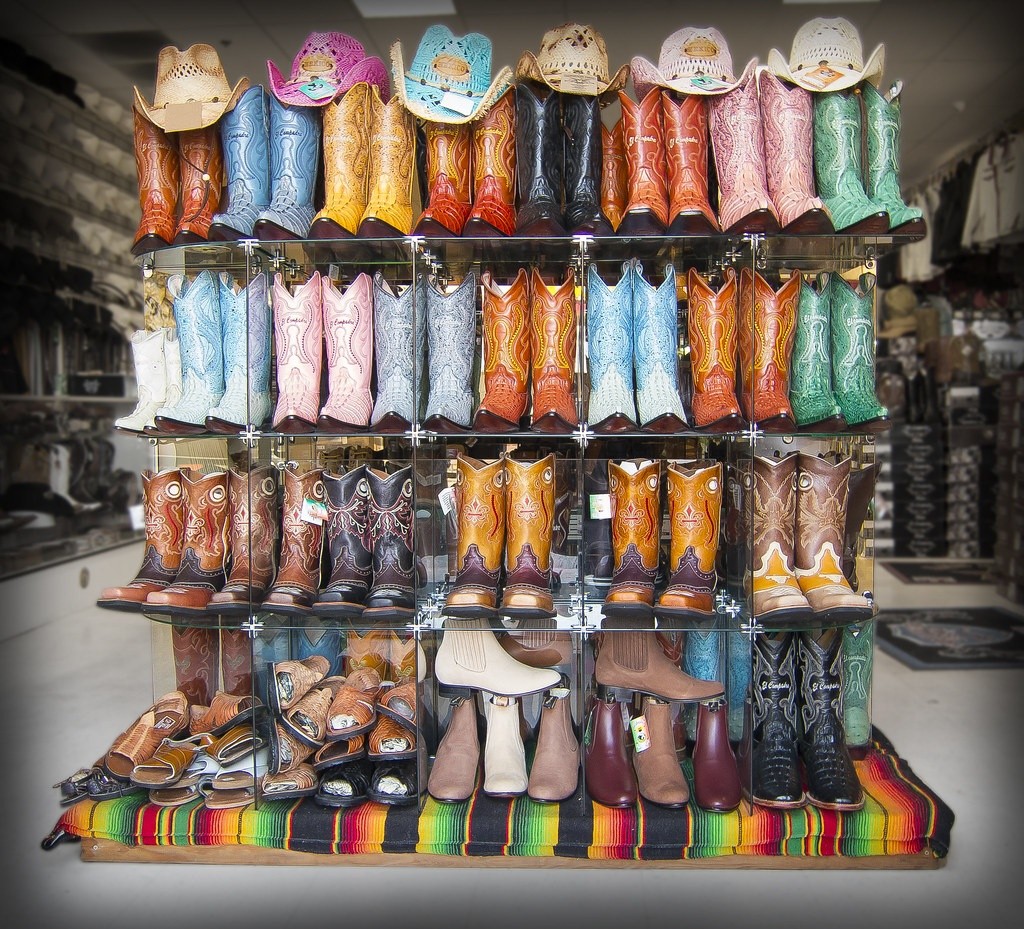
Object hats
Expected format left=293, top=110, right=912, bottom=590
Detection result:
left=391, top=24, right=514, bottom=125
left=770, top=17, right=885, bottom=92
left=133, top=43, right=248, bottom=136
left=631, top=26, right=759, bottom=95
left=518, top=23, right=630, bottom=94
left=268, top=30, right=391, bottom=108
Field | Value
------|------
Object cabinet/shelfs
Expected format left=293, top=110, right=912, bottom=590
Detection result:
left=36, top=233, right=955, bottom=870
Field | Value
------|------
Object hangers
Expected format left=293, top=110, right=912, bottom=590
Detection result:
left=904, top=120, right=1015, bottom=202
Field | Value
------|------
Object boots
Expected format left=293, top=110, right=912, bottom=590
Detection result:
left=95, top=69, right=931, bottom=810
